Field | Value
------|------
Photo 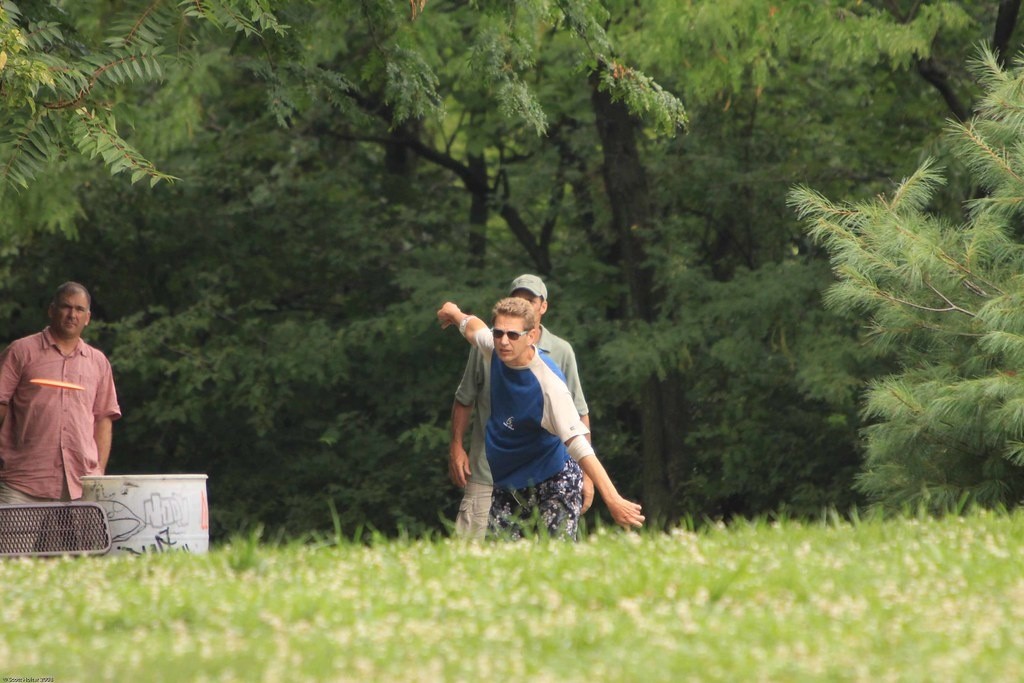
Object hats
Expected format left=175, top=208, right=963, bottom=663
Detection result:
left=508, top=273, right=547, bottom=301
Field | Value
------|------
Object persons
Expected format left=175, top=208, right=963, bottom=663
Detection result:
left=437, top=274, right=645, bottom=544
left=0, top=282, right=121, bottom=554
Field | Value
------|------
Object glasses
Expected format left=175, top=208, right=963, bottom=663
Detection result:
left=491, top=328, right=528, bottom=340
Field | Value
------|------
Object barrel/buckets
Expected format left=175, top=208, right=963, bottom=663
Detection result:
left=79, top=474, right=211, bottom=556
